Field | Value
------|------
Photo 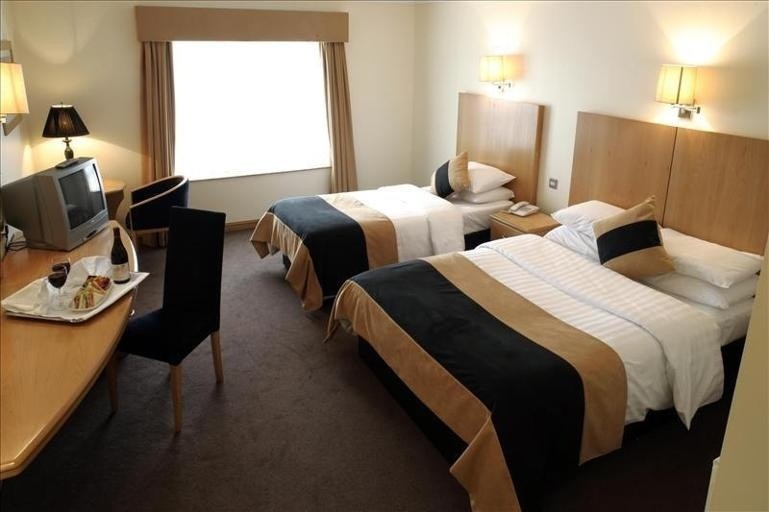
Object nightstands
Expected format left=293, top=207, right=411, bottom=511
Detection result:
left=488, top=209, right=563, bottom=240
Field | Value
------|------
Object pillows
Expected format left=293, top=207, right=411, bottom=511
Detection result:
left=453, top=186, right=516, bottom=204
left=464, top=160, right=517, bottom=195
left=543, top=224, right=600, bottom=261
left=642, top=270, right=760, bottom=311
left=591, top=194, right=676, bottom=280
left=430, top=151, right=470, bottom=198
left=659, top=226, right=765, bottom=289
left=550, top=198, right=661, bottom=243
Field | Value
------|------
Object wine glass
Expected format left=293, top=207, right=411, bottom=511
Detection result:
left=46, top=254, right=79, bottom=314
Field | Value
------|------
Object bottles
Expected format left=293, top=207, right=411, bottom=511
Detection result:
left=106, top=224, right=131, bottom=284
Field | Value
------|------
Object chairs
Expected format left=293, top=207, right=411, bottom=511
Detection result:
left=107, top=208, right=226, bottom=433
left=125, top=175, right=189, bottom=250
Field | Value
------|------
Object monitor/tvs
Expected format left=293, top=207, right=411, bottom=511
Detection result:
left=0, top=157, right=109, bottom=251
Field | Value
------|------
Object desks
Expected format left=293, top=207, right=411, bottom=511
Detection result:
left=0, top=219, right=138, bottom=477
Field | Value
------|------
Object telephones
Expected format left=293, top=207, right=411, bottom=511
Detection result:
left=509, top=200, right=539, bottom=217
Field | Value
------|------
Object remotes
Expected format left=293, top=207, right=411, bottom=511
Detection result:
left=55, top=158, right=79, bottom=169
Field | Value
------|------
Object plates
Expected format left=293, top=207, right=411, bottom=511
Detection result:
left=69, top=279, right=117, bottom=314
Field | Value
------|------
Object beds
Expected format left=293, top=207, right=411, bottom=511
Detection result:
left=249, top=92, right=545, bottom=313
left=322, top=110, right=769, bottom=512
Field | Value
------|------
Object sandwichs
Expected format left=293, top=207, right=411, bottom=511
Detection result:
left=73, top=282, right=107, bottom=309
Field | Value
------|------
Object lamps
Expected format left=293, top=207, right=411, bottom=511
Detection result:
left=41, top=102, right=89, bottom=159
left=0, top=62, right=30, bottom=114
left=656, top=64, right=700, bottom=120
left=476, top=55, right=511, bottom=93
